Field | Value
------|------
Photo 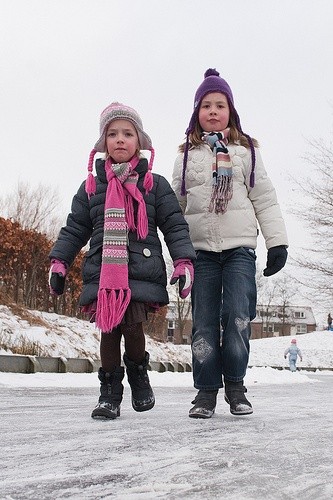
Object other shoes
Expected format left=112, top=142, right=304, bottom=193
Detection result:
left=188, top=393, right=217, bottom=418
left=224, top=383, right=254, bottom=416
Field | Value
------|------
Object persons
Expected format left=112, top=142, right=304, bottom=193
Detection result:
left=172, top=69, right=289, bottom=419
left=49, top=102, right=197, bottom=420
left=327, top=314, right=333, bottom=328
left=284, top=338, right=303, bottom=372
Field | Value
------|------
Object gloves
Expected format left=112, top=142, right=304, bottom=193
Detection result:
left=263, top=248, right=288, bottom=277
left=170, top=260, right=195, bottom=299
left=48, top=259, right=67, bottom=297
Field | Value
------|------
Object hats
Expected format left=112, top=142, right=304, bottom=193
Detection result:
left=291, top=339, right=297, bottom=344
left=179, top=67, right=255, bottom=196
left=83, top=102, right=156, bottom=201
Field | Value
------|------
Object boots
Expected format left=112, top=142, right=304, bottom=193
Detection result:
left=123, top=351, right=155, bottom=411
left=90, top=367, right=125, bottom=420
